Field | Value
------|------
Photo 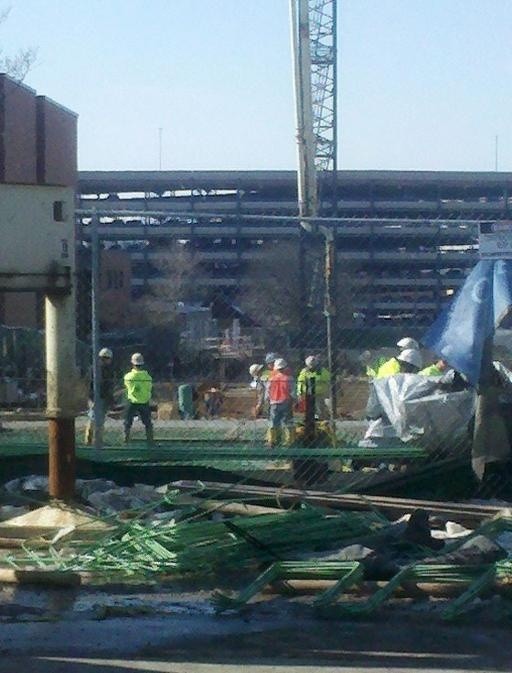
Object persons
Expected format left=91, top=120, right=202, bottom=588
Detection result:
left=201, top=386, right=224, bottom=419
left=119, top=349, right=156, bottom=446
left=246, top=336, right=454, bottom=446
left=80, top=346, right=114, bottom=449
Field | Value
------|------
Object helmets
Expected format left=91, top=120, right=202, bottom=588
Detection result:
left=394, top=336, right=452, bottom=371
left=99, top=348, right=113, bottom=359
left=250, top=352, right=319, bottom=376
left=131, top=353, right=145, bottom=365
